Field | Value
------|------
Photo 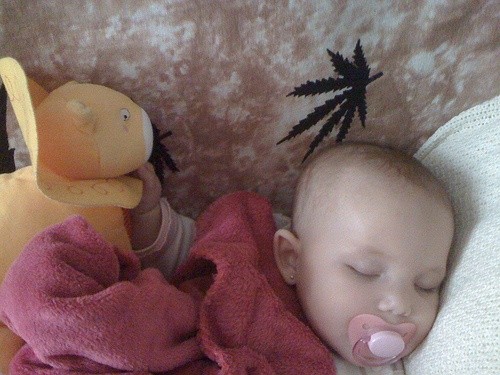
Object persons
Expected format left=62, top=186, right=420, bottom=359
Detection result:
left=127, top=139, right=456, bottom=375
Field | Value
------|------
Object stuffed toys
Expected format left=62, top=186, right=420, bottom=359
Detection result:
left=1, top=57, right=157, bottom=375
